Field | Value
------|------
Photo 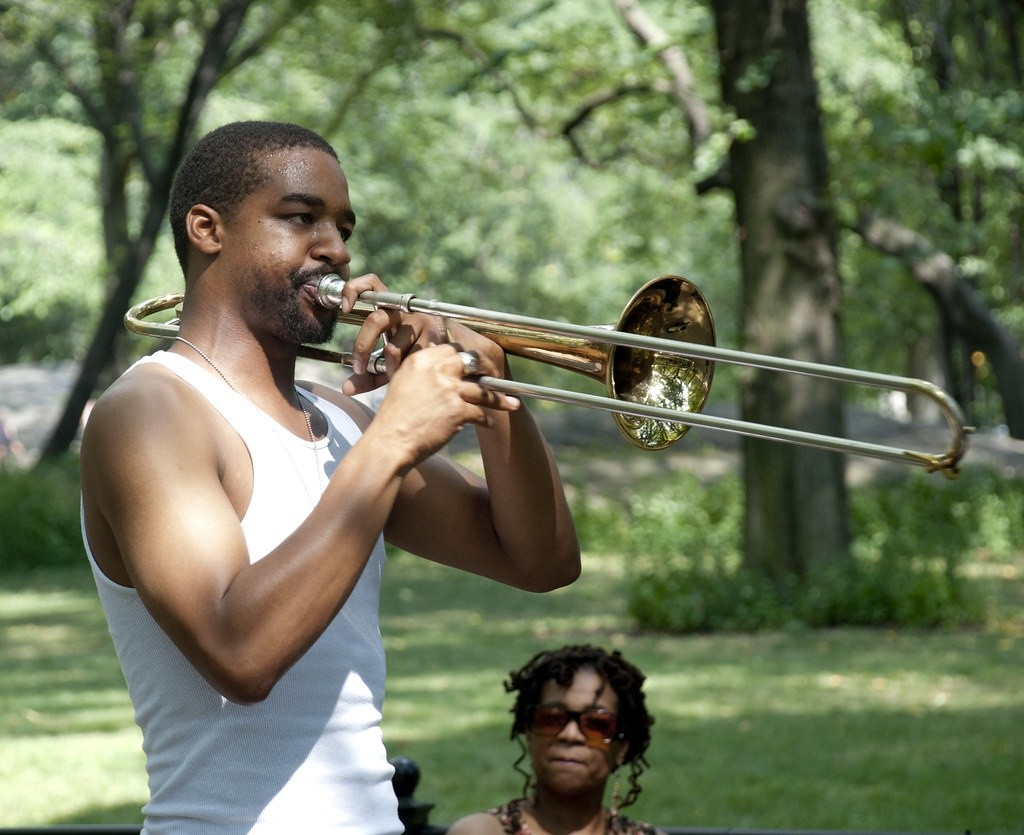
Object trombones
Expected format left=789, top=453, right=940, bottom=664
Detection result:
left=125, top=270, right=979, bottom=474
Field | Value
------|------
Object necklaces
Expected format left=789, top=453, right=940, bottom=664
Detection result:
left=174, top=336, right=321, bottom=509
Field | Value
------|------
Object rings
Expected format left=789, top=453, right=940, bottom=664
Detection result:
left=457, top=350, right=480, bottom=374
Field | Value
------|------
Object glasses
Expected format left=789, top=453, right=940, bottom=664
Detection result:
left=527, top=705, right=619, bottom=741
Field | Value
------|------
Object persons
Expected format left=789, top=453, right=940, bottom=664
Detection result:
left=77, top=119, right=582, bottom=834
left=446, top=643, right=666, bottom=835
